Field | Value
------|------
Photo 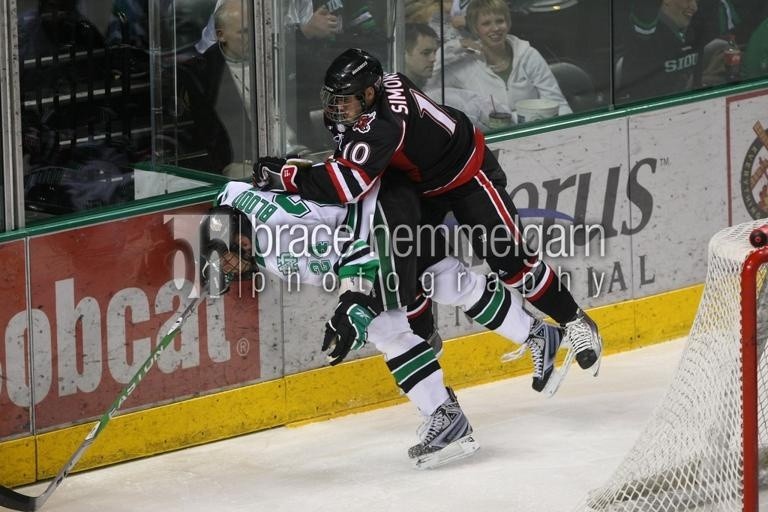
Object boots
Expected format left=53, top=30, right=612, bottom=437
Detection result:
left=562, top=305, right=601, bottom=371
left=406, top=385, right=474, bottom=460
left=527, top=318, right=565, bottom=393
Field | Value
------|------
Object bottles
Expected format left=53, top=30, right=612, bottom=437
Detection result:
left=722, top=32, right=740, bottom=80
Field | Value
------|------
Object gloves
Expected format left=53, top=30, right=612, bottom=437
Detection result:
left=320, top=290, right=384, bottom=370
left=251, top=155, right=286, bottom=189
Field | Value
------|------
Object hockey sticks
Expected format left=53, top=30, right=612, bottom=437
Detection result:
left=0, top=281, right=215, bottom=511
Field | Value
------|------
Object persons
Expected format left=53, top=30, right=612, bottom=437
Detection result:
left=197, top=176, right=564, bottom=458
left=253, top=51, right=602, bottom=370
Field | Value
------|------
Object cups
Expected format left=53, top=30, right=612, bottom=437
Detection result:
left=487, top=111, right=512, bottom=129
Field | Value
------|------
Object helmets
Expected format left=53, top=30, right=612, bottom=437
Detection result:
left=321, top=46, right=383, bottom=125
left=197, top=204, right=259, bottom=280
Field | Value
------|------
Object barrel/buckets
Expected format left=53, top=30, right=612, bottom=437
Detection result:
left=515, top=99, right=560, bottom=125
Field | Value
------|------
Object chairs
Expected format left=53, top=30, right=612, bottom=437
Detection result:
left=609, top=50, right=632, bottom=111
left=546, top=56, right=603, bottom=117
